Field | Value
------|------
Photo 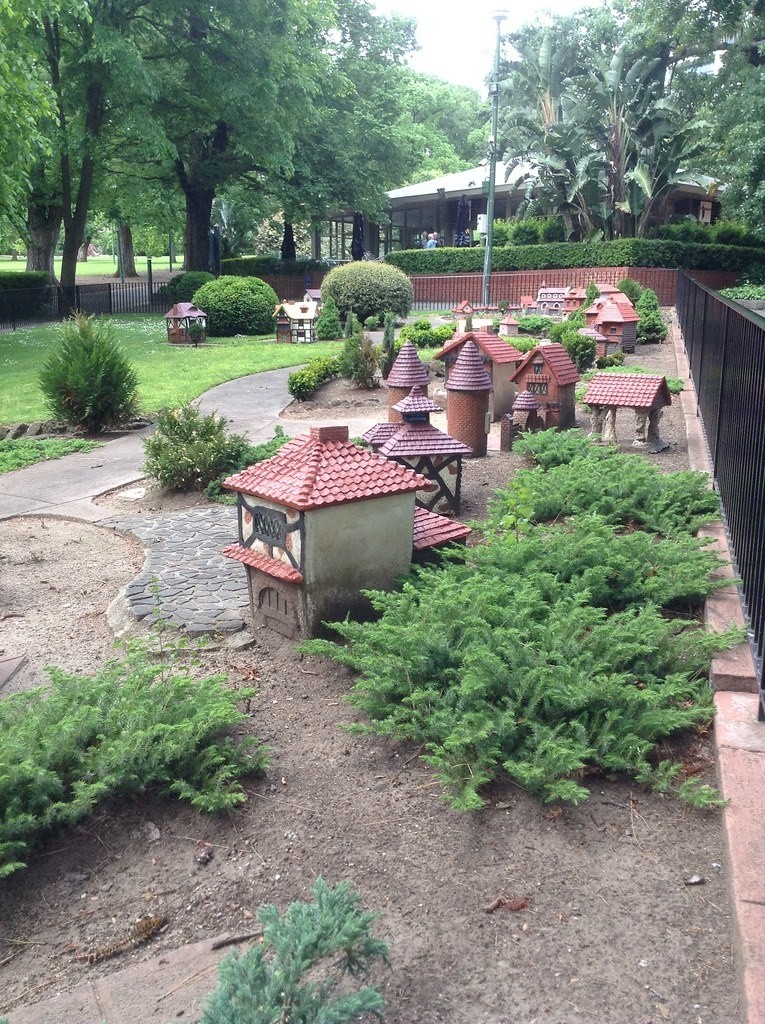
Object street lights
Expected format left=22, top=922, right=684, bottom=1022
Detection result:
left=482, top=4, right=510, bottom=307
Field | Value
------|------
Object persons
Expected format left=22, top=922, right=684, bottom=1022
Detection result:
left=418, top=230, right=442, bottom=248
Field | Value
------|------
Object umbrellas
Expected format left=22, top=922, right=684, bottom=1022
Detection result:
left=349, top=210, right=365, bottom=261
left=454, top=193, right=471, bottom=247
